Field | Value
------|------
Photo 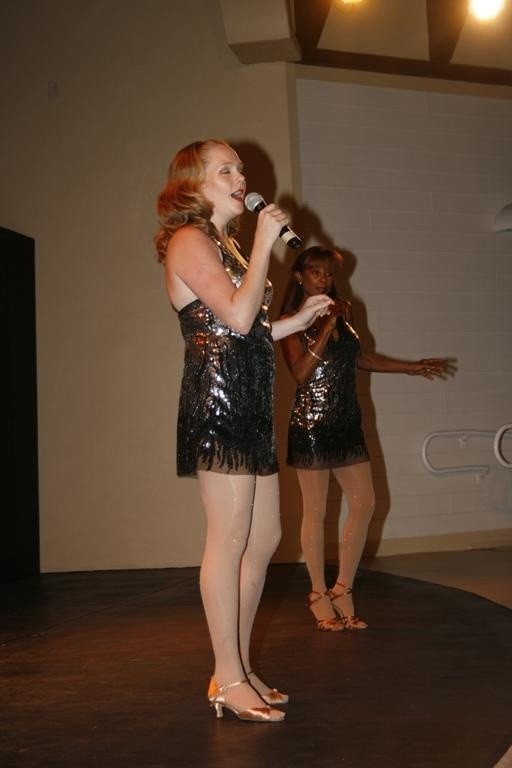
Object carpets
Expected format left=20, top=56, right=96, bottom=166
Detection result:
left=0, top=562, right=512, bottom=768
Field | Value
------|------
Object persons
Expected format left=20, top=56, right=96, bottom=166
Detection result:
left=277, top=246, right=444, bottom=632
left=155, top=140, right=335, bottom=722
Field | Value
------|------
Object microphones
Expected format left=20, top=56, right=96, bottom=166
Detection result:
left=244, top=192, right=302, bottom=249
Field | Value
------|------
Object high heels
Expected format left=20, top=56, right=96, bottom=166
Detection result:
left=208, top=670, right=289, bottom=721
left=307, top=582, right=368, bottom=632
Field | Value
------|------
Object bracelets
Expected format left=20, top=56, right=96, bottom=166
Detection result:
left=308, top=348, right=323, bottom=361
